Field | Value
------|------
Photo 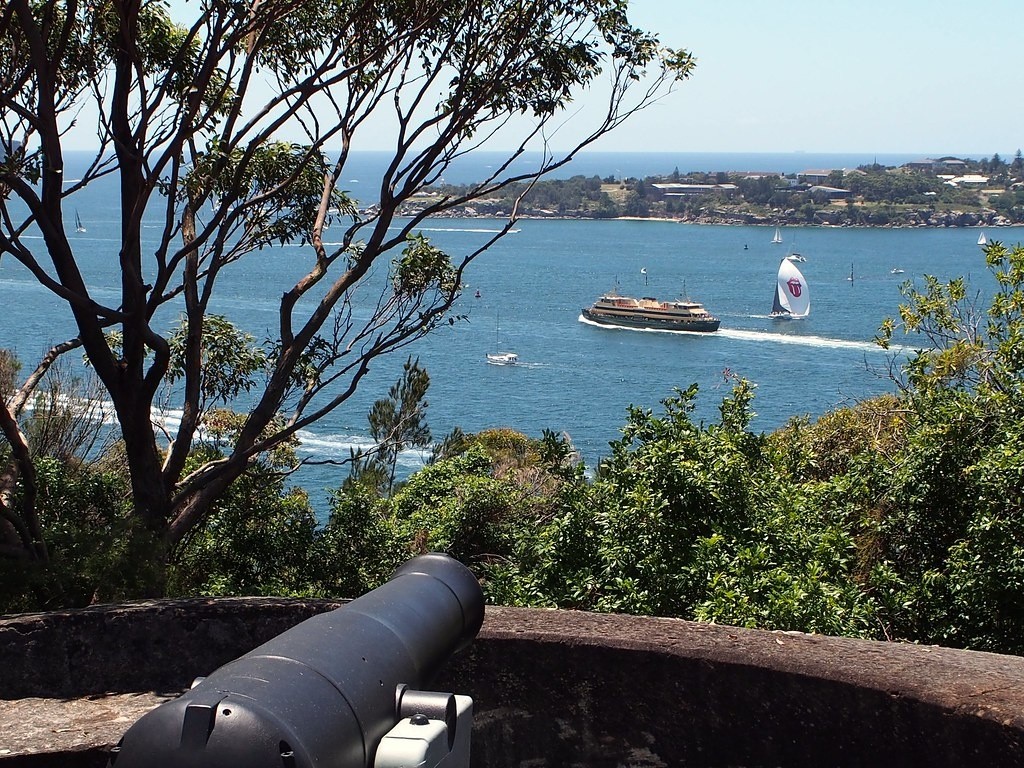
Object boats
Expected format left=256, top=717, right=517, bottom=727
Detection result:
left=891, top=269, right=905, bottom=274
left=781, top=253, right=806, bottom=263
left=581, top=291, right=721, bottom=333
left=507, top=228, right=521, bottom=234
left=474, top=289, right=481, bottom=297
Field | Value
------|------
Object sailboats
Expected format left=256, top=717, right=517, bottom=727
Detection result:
left=485, top=310, right=518, bottom=364
left=977, top=232, right=986, bottom=244
left=768, top=258, right=810, bottom=320
left=771, top=227, right=783, bottom=243
left=75, top=210, right=87, bottom=233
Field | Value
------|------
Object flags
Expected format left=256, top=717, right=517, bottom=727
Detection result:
left=641, top=268, right=646, bottom=273
